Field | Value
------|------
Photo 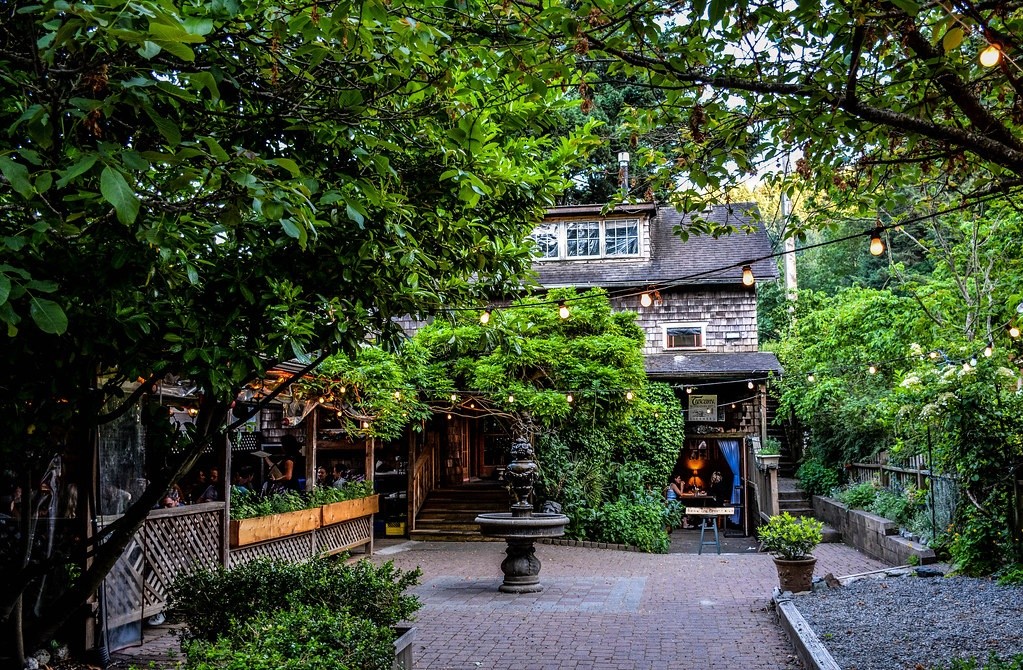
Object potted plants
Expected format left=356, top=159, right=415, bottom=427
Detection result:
left=125, top=551, right=424, bottom=670
left=755, top=435, right=782, bottom=470
left=230, top=479, right=321, bottom=546
left=305, top=480, right=381, bottom=526
left=755, top=511, right=824, bottom=593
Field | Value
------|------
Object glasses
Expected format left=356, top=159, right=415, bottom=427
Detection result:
left=200, top=474, right=204, bottom=477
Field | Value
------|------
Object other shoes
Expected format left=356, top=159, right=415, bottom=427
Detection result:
left=683, top=523, right=694, bottom=528
left=698, top=523, right=707, bottom=527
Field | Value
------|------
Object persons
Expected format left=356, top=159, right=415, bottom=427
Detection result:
left=666, top=474, right=694, bottom=528
left=698, top=471, right=726, bottom=527
left=332, top=463, right=349, bottom=488
left=195, top=467, right=217, bottom=504
left=238, top=465, right=254, bottom=492
left=190, top=468, right=208, bottom=502
left=316, top=466, right=332, bottom=487
left=158, top=487, right=185, bottom=509
left=268, top=433, right=303, bottom=494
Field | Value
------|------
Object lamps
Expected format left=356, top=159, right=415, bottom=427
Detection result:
left=248, top=377, right=262, bottom=405
left=188, top=401, right=200, bottom=424
left=137, top=374, right=159, bottom=395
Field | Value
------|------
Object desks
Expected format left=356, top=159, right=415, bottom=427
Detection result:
left=679, top=495, right=716, bottom=506
left=723, top=503, right=745, bottom=538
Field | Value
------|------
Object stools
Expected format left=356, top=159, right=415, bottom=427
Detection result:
left=718, top=500, right=733, bottom=531
left=698, top=515, right=721, bottom=555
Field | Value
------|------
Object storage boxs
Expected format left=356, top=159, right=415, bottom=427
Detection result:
left=386, top=522, right=408, bottom=536
